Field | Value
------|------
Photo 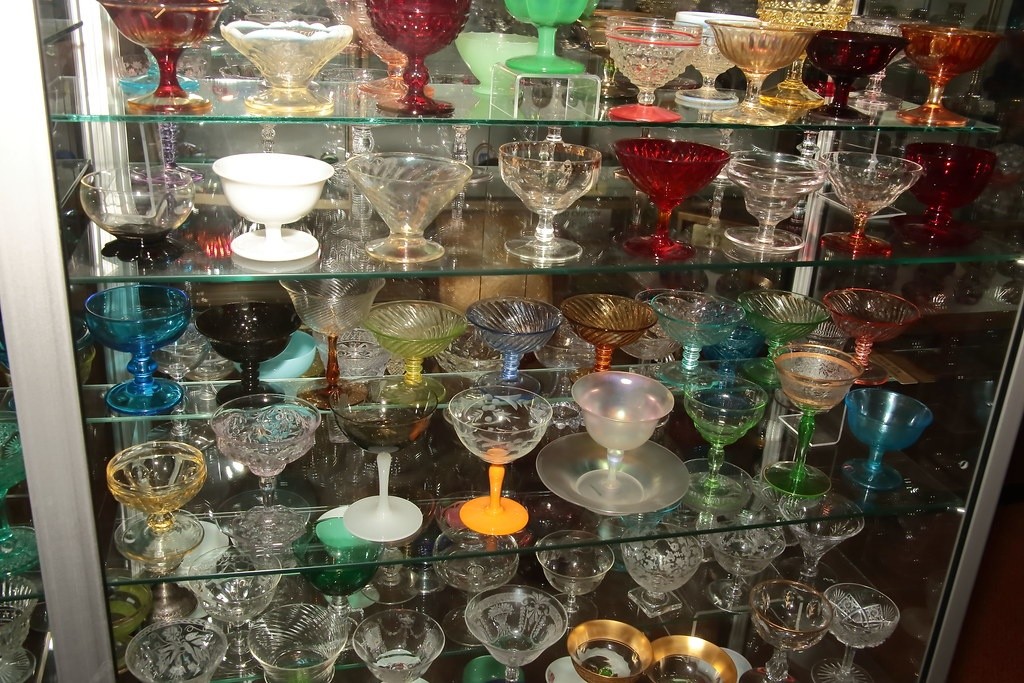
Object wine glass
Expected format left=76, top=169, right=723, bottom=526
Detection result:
left=2, top=1, right=1013, bottom=682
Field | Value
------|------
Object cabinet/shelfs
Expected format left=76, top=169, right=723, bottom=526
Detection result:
left=0, top=0, right=1024, bottom=683
